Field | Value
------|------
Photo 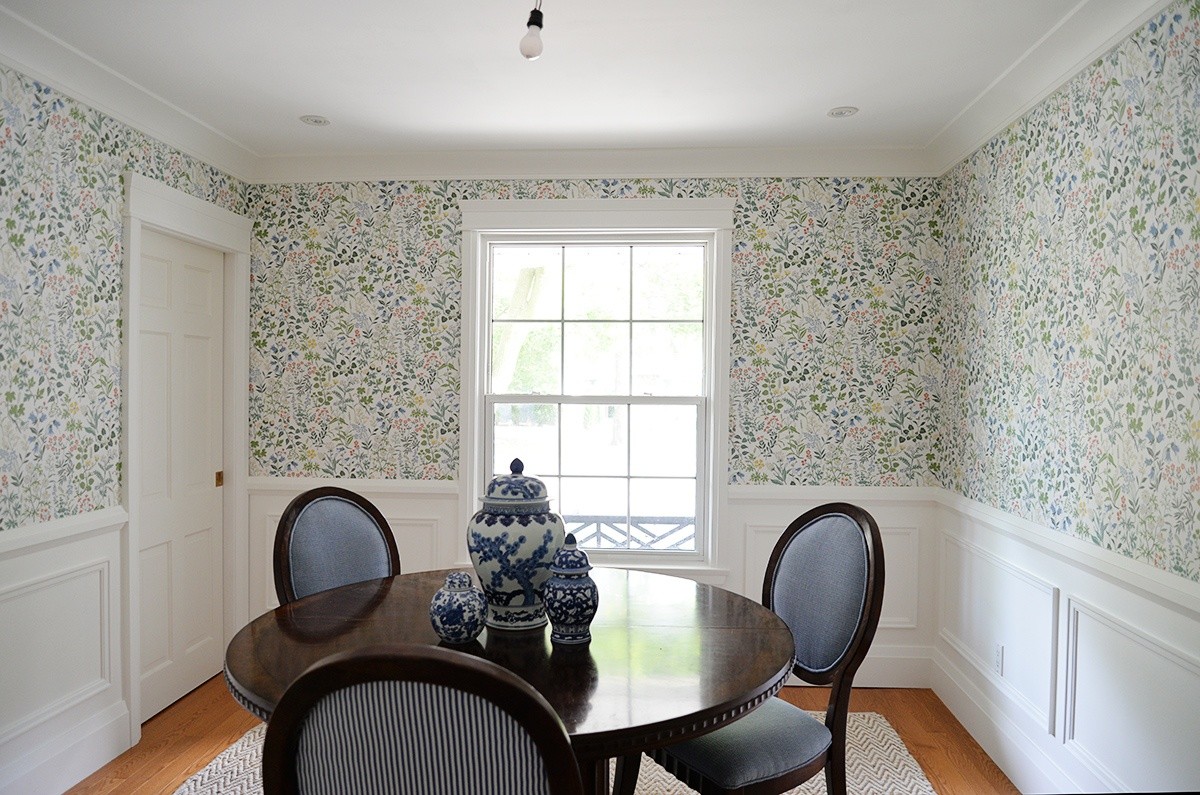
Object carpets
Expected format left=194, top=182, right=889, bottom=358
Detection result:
left=173, top=710, right=937, bottom=795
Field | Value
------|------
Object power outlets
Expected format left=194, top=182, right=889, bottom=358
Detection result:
left=994, top=640, right=1004, bottom=677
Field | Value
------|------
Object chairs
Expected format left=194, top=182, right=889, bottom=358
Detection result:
left=612, top=502, right=885, bottom=795
left=262, top=641, right=586, bottom=795
left=272, top=485, right=402, bottom=607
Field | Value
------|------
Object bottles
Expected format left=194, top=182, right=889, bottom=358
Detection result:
left=429, top=571, right=488, bottom=643
left=466, top=459, right=566, bottom=630
left=543, top=533, right=599, bottom=644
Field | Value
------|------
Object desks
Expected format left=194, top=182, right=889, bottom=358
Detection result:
left=224, top=566, right=798, bottom=795
